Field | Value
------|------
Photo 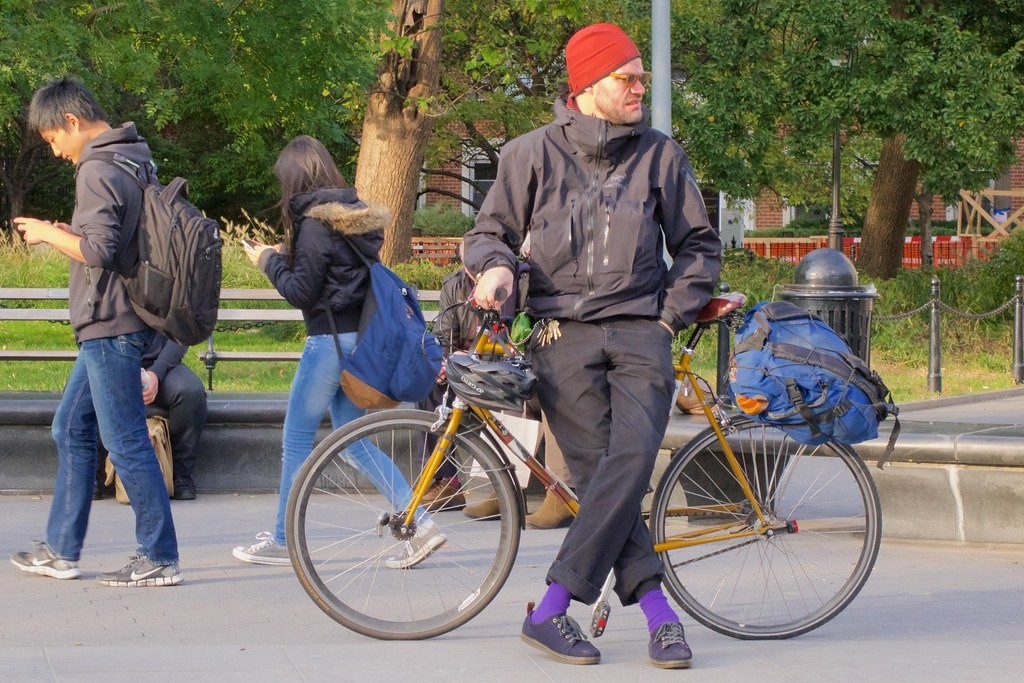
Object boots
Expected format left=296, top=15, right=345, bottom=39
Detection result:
left=526, top=489, right=576, bottom=530
left=463, top=491, right=528, bottom=520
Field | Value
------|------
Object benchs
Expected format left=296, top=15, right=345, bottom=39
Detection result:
left=0, top=289, right=1024, bottom=545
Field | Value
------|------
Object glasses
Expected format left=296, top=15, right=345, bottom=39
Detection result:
left=609, top=72, right=650, bottom=87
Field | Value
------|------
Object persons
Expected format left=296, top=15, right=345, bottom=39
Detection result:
left=420, top=238, right=530, bottom=529
left=232, top=136, right=447, bottom=568
left=92, top=327, right=207, bottom=499
left=461, top=22, right=721, bottom=669
left=462, top=408, right=575, bottom=529
left=9, top=78, right=185, bottom=587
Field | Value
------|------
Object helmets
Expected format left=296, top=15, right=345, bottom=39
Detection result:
left=446, top=320, right=539, bottom=414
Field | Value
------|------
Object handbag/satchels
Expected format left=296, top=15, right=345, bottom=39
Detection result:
left=676, top=372, right=718, bottom=416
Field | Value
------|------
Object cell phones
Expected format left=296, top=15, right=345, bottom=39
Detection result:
left=242, top=239, right=256, bottom=251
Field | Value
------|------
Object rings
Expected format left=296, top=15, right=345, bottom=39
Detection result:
left=153, top=395, right=156, bottom=398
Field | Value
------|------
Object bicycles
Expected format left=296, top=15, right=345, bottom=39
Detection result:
left=287, top=266, right=885, bottom=638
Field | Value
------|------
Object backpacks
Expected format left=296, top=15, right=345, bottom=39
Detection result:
left=104, top=415, right=174, bottom=504
left=323, top=224, right=443, bottom=410
left=723, top=299, right=901, bottom=470
left=74, top=150, right=224, bottom=349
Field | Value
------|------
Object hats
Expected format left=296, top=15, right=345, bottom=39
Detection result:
left=566, top=22, right=641, bottom=96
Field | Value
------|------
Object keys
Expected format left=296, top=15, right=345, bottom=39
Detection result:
left=536, top=320, right=561, bottom=346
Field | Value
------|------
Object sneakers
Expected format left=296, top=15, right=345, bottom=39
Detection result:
left=233, top=531, right=293, bottom=565
left=174, top=475, right=197, bottom=500
left=521, top=602, right=601, bottom=664
left=96, top=554, right=184, bottom=587
left=92, top=472, right=103, bottom=500
left=648, top=621, right=692, bottom=668
left=10, top=539, right=82, bottom=579
left=419, top=477, right=467, bottom=514
left=386, top=522, right=448, bottom=569
